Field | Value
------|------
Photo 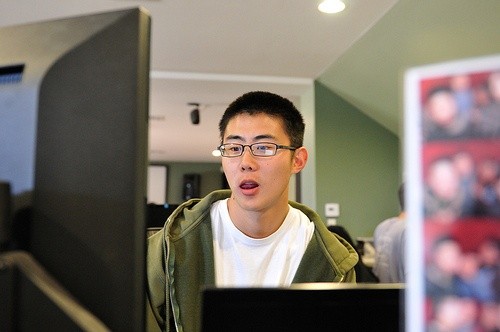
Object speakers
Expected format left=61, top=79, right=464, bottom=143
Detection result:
left=183, top=174, right=201, bottom=202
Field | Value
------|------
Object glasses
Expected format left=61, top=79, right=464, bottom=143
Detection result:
left=217, top=142, right=297, bottom=157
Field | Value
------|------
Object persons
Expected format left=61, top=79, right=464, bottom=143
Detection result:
left=373, top=183, right=408, bottom=283
left=420, top=70, right=500, bottom=332
left=147, top=90, right=359, bottom=332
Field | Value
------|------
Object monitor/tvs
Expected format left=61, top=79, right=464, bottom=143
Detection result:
left=0, top=7, right=151, bottom=332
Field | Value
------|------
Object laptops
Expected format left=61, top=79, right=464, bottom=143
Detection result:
left=199, top=284, right=406, bottom=332
